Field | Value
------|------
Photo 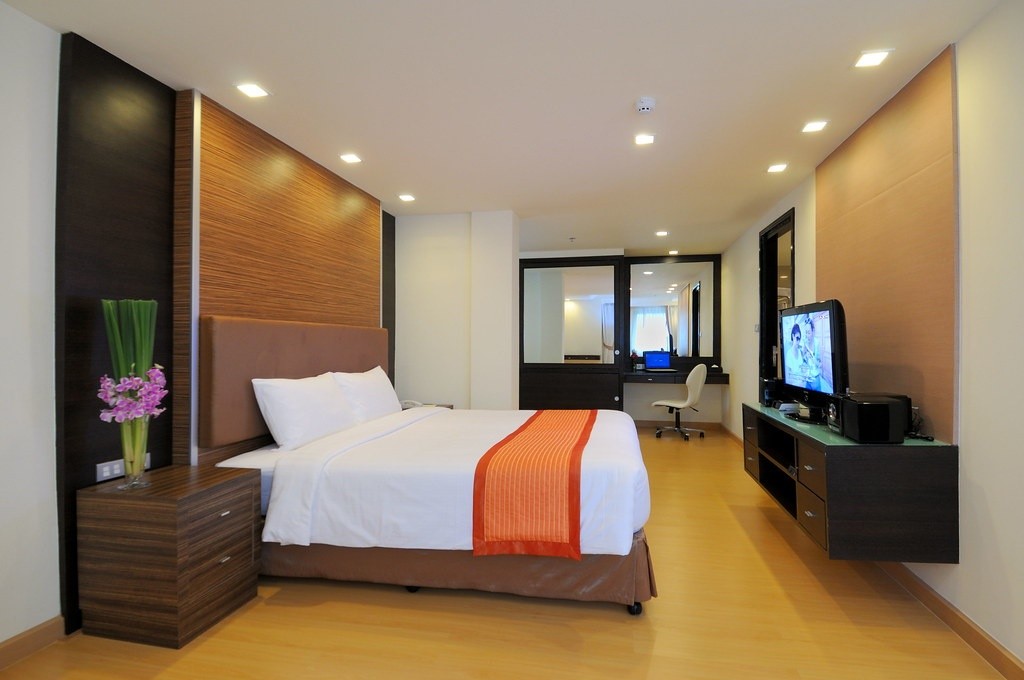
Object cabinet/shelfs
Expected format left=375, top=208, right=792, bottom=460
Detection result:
left=741, top=401, right=961, bottom=564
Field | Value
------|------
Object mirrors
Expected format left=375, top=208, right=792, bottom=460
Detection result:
left=625, top=254, right=721, bottom=365
left=518, top=259, right=620, bottom=368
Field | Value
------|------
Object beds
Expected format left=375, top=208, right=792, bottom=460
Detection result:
left=198, top=315, right=659, bottom=617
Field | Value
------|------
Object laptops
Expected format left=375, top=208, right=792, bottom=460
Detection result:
left=643, top=351, right=678, bottom=372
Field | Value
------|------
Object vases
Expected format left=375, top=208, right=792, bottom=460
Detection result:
left=117, top=414, right=150, bottom=491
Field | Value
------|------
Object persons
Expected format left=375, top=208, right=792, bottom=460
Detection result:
left=785, top=317, right=822, bottom=391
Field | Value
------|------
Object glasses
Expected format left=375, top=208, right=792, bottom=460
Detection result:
left=793, top=337, right=800, bottom=341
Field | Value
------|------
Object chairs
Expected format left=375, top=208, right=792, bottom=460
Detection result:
left=652, top=363, right=707, bottom=440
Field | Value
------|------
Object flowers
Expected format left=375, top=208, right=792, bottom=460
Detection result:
left=97, top=298, right=168, bottom=472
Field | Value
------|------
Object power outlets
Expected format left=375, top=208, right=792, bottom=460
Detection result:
left=97, top=453, right=150, bottom=482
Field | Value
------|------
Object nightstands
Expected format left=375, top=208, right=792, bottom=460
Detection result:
left=76, top=464, right=261, bottom=649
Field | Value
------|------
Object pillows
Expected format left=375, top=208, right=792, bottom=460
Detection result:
left=251, top=371, right=355, bottom=449
left=334, top=366, right=403, bottom=424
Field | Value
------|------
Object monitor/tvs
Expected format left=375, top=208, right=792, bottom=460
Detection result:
left=780, top=299, right=849, bottom=425
